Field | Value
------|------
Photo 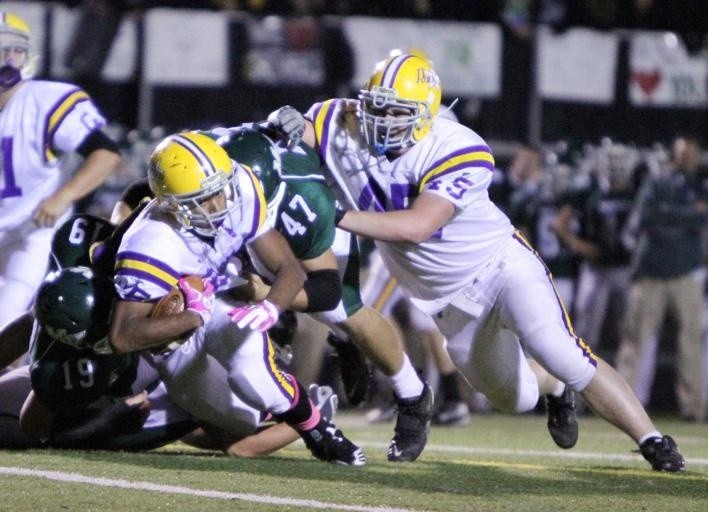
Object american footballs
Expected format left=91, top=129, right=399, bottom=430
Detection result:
left=149, top=275, right=205, bottom=355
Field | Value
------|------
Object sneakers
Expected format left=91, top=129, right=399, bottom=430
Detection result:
left=386, top=381, right=435, bottom=461
left=543, top=383, right=580, bottom=446
left=640, top=435, right=684, bottom=471
left=302, top=417, right=362, bottom=461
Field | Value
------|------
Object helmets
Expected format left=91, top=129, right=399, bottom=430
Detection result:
left=354, top=50, right=442, bottom=157
left=33, top=265, right=110, bottom=348
left=217, top=127, right=281, bottom=206
left=0, top=11, right=37, bottom=84
left=147, top=129, right=245, bottom=238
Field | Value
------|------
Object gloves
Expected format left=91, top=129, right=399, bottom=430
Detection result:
left=266, top=105, right=305, bottom=150
left=178, top=275, right=214, bottom=323
left=226, top=297, right=279, bottom=334
left=308, top=384, right=339, bottom=418
